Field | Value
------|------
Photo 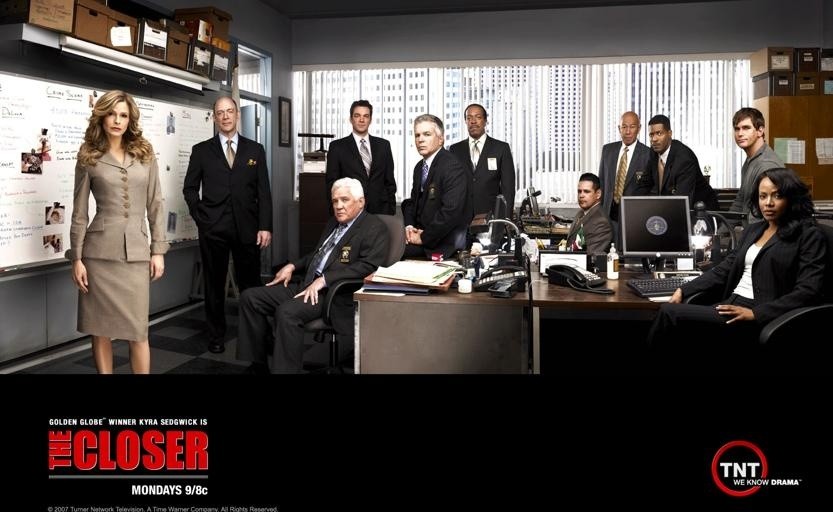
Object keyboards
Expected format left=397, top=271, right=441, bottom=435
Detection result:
left=626, top=279, right=694, bottom=297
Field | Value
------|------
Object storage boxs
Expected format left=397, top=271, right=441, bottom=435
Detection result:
left=172, top=6, right=233, bottom=41
left=209, top=47, right=232, bottom=87
left=0, top=0, right=74, bottom=34
left=750, top=47, right=833, bottom=98
left=186, top=37, right=213, bottom=77
left=166, top=28, right=190, bottom=70
left=73, top=0, right=107, bottom=47
left=107, top=8, right=138, bottom=55
left=137, top=16, right=168, bottom=64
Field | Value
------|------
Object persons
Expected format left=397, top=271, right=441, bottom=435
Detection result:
left=401, top=103, right=516, bottom=261
left=564, top=110, right=723, bottom=273
left=64, top=86, right=171, bottom=374
left=325, top=98, right=397, bottom=218
left=716, top=105, right=786, bottom=250
left=20, top=154, right=41, bottom=174
left=235, top=177, right=392, bottom=374
left=47, top=210, right=59, bottom=225
left=469, top=241, right=484, bottom=280
left=49, top=235, right=61, bottom=253
left=640, top=167, right=831, bottom=377
left=182, top=96, right=275, bottom=355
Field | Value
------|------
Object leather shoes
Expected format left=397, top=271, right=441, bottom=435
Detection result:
left=206, top=342, right=226, bottom=354
left=249, top=360, right=271, bottom=376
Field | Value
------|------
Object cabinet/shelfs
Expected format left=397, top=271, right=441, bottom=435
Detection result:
left=749, top=97, right=833, bottom=204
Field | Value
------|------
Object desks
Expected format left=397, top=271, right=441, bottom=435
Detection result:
left=350, top=258, right=532, bottom=374
left=523, top=224, right=572, bottom=235
left=531, top=263, right=704, bottom=375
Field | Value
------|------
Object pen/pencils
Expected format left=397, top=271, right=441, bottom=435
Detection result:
left=538, top=239, right=546, bottom=249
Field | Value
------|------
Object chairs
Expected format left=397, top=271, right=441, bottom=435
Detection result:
left=271, top=214, right=407, bottom=375
left=189, top=257, right=241, bottom=302
left=683, top=213, right=833, bottom=345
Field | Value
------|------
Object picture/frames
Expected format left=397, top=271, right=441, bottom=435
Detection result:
left=278, top=95, right=290, bottom=147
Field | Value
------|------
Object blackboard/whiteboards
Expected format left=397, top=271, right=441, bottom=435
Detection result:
left=0, top=69, right=215, bottom=284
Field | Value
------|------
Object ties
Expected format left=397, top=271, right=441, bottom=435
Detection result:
left=472, top=141, right=480, bottom=168
left=614, top=148, right=629, bottom=203
left=304, top=223, right=346, bottom=286
left=421, top=161, right=428, bottom=186
left=360, top=139, right=371, bottom=175
left=657, top=158, right=663, bottom=191
left=224, top=140, right=236, bottom=169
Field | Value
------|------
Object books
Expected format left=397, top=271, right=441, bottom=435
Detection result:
left=521, top=215, right=563, bottom=234
left=362, top=266, right=457, bottom=293
left=811, top=200, right=833, bottom=212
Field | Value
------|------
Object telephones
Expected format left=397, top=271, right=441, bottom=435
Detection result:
left=473, top=265, right=529, bottom=290
left=546, top=263, right=607, bottom=289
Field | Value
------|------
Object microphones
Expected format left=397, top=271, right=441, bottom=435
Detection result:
left=524, top=190, right=542, bottom=200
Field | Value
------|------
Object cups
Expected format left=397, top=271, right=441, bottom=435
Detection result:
left=458, top=278, right=472, bottom=294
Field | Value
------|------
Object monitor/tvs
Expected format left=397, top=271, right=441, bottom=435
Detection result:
left=527, top=186, right=541, bottom=216
left=621, top=195, right=694, bottom=280
left=487, top=194, right=512, bottom=256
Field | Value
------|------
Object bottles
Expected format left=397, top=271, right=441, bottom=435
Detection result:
left=607, top=243, right=619, bottom=280
